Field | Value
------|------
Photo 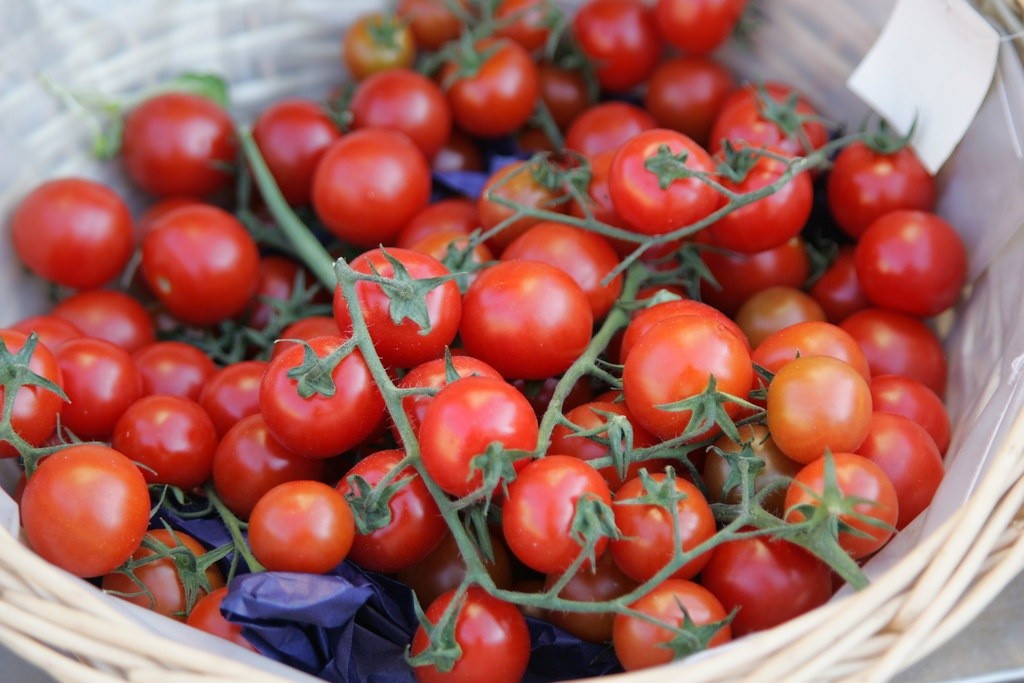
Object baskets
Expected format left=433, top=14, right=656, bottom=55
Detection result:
left=0, top=0, right=1024, bottom=683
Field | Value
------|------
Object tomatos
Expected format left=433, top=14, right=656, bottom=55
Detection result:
left=0, top=1, right=970, bottom=683
